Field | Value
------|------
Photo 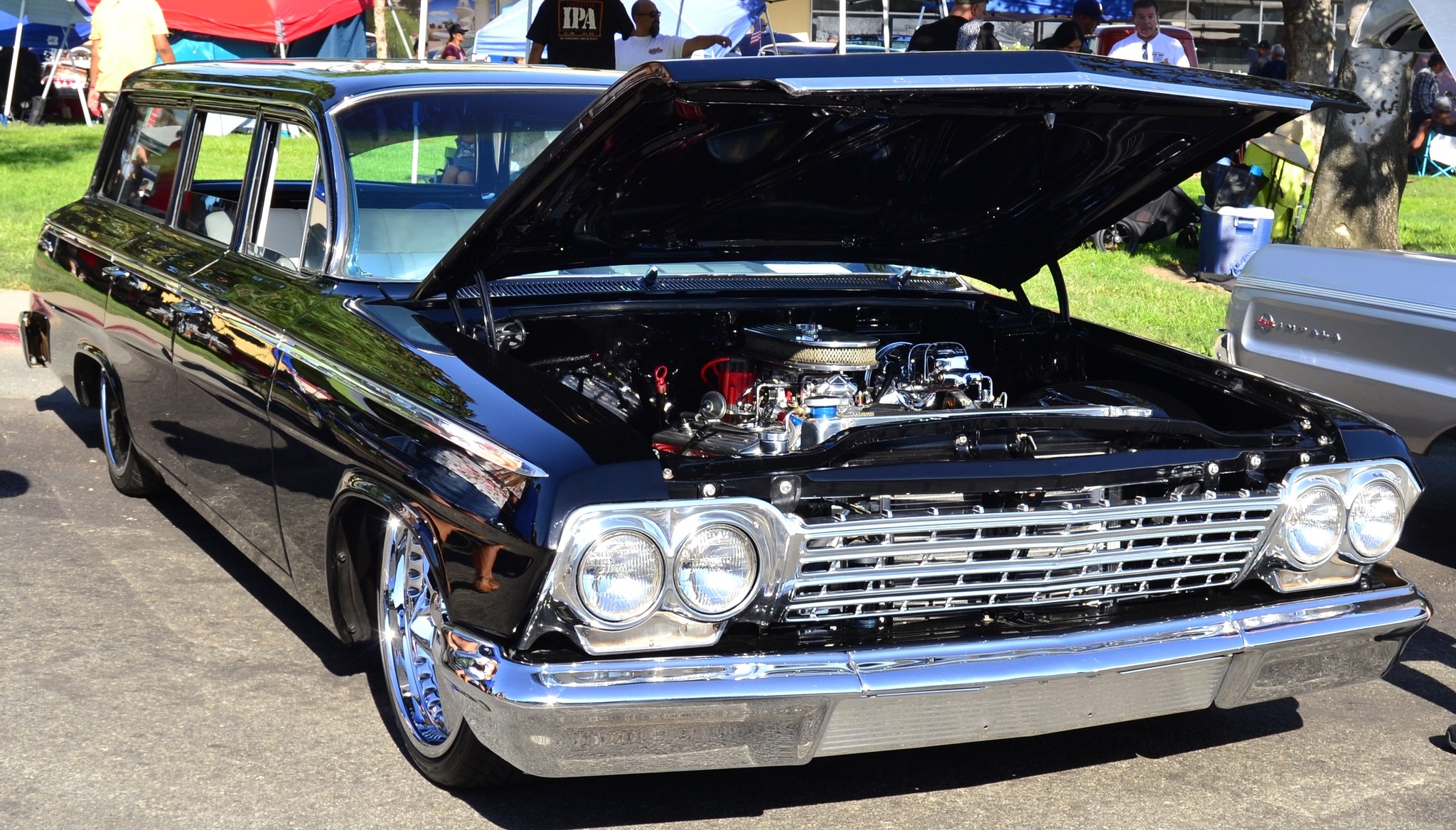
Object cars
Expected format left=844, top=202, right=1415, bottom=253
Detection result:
left=21, top=32, right=1436, bottom=791
left=1211, top=0, right=1455, bottom=564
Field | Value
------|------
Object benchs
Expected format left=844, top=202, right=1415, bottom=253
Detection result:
left=205, top=208, right=487, bottom=280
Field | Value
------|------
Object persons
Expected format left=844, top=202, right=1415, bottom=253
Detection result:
left=440, top=24, right=466, bottom=60
left=615, top=0, right=733, bottom=71
left=1407, top=52, right=1456, bottom=174
left=1071, top=0, right=1100, bottom=55
left=1033, top=20, right=1095, bottom=55
left=982, top=22, right=995, bottom=35
left=908, top=0, right=1003, bottom=52
left=1237, top=35, right=1290, bottom=80
left=410, top=40, right=430, bottom=59
left=88, top=0, right=177, bottom=111
left=526, top=0, right=637, bottom=69
left=1108, top=0, right=1191, bottom=68
left=440, top=133, right=476, bottom=185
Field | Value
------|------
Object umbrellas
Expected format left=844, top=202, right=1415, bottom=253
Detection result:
left=0, top=0, right=92, bottom=127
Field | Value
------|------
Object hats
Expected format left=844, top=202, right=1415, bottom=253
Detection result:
left=1074, top=0, right=1103, bottom=21
left=1258, top=40, right=1271, bottom=49
left=760, top=18, right=768, bottom=26
left=450, top=24, right=468, bottom=34
left=955, top=0, right=988, bottom=4
left=1428, top=96, right=1452, bottom=111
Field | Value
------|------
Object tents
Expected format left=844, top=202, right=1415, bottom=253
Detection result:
left=475, top=0, right=768, bottom=60
left=153, top=0, right=374, bottom=63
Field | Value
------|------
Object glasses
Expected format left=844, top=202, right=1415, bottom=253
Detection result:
left=1142, top=41, right=1148, bottom=60
left=634, top=10, right=661, bottom=18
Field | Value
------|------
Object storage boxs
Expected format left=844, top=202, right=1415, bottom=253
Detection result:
left=1196, top=204, right=1274, bottom=276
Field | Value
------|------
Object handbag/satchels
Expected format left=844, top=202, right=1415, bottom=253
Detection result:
left=1201, top=156, right=1269, bottom=211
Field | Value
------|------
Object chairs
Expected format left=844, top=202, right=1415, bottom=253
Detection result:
left=1407, top=125, right=1456, bottom=179
